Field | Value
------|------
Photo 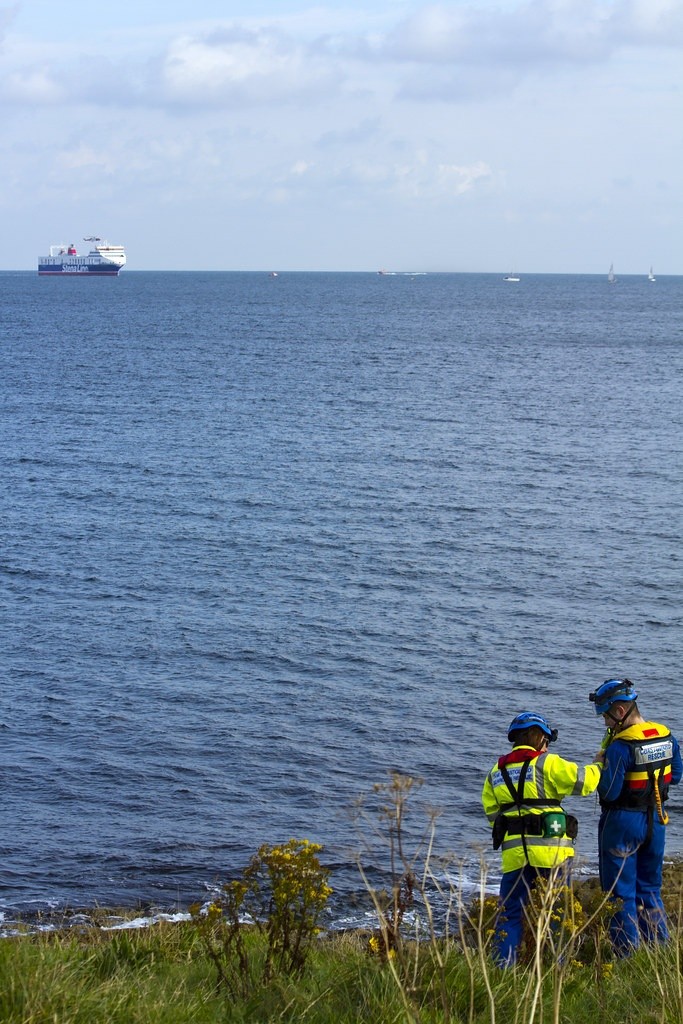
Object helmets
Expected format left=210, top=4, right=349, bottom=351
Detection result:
left=589, top=679, right=638, bottom=715
left=506, top=712, right=558, bottom=743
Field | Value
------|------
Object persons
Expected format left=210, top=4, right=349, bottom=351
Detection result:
left=479, top=708, right=605, bottom=970
left=588, top=676, right=683, bottom=963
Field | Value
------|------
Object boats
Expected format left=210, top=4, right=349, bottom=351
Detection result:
left=37, top=235, right=127, bottom=276
left=503, top=276, right=520, bottom=282
